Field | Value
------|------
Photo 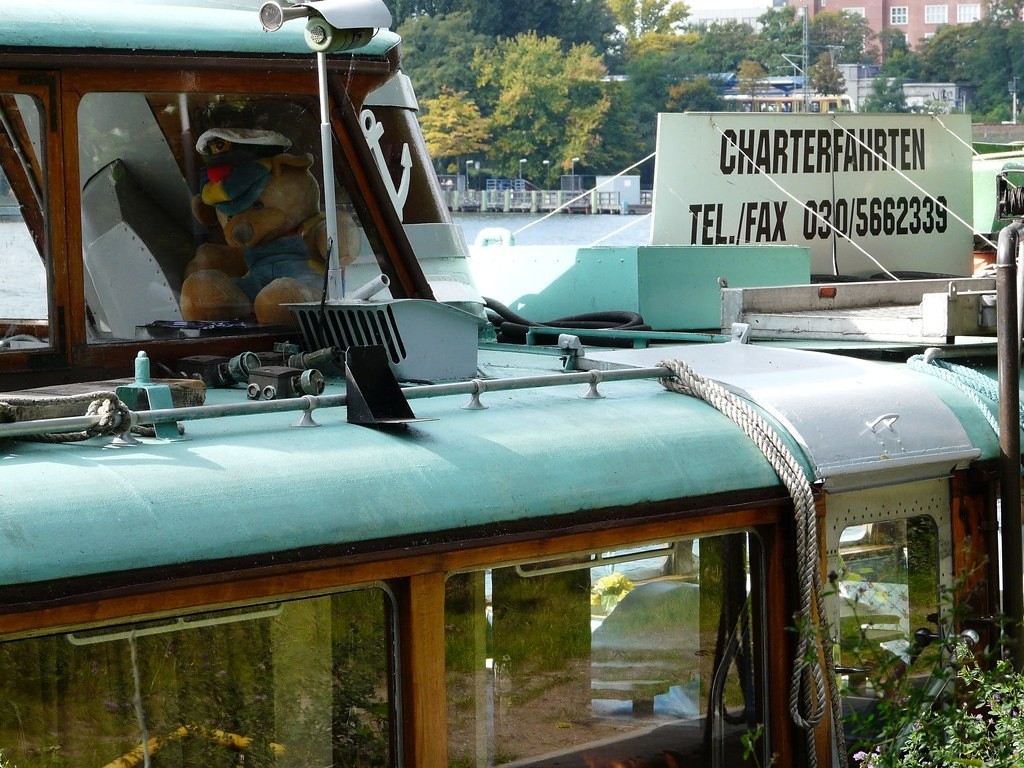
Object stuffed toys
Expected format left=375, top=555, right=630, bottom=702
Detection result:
left=180, top=128, right=362, bottom=326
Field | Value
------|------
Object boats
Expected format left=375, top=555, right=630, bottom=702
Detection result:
left=0, top=2, right=1024, bottom=767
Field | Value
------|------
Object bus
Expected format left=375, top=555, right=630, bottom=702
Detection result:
left=724, top=91, right=857, bottom=112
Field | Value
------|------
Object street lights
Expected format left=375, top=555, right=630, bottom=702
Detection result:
left=466, top=160, right=474, bottom=191
left=542, top=159, right=550, bottom=190
left=571, top=157, right=579, bottom=193
left=519, top=158, right=527, bottom=191
